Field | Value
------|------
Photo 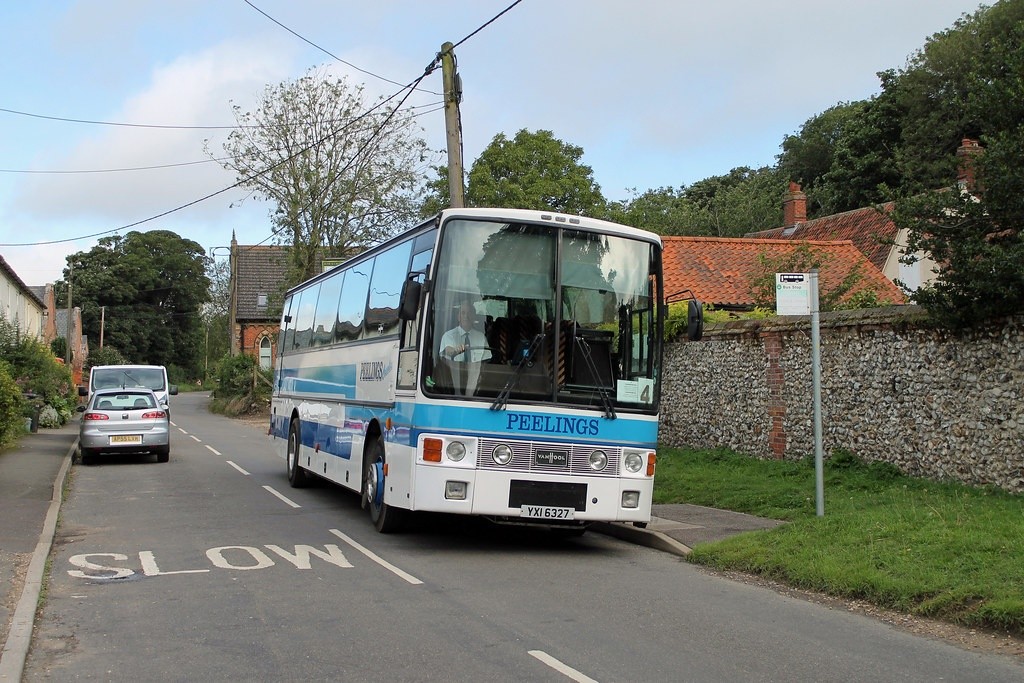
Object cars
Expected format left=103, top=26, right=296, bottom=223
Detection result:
left=75, top=387, right=170, bottom=464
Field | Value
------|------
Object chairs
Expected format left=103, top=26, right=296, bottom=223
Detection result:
left=476, top=313, right=581, bottom=389
left=99, top=400, right=111, bottom=408
left=134, top=399, right=148, bottom=407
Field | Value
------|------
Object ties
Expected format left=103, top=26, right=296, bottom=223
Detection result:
left=465, top=333, right=474, bottom=364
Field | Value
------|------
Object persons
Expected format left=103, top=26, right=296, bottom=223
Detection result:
left=438, top=303, right=493, bottom=364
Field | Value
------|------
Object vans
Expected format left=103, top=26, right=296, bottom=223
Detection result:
left=79, top=363, right=178, bottom=421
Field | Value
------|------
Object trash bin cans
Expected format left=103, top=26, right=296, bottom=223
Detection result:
left=23, top=395, right=39, bottom=433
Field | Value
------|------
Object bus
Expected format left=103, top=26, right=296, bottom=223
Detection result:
left=268, top=207, right=704, bottom=535
